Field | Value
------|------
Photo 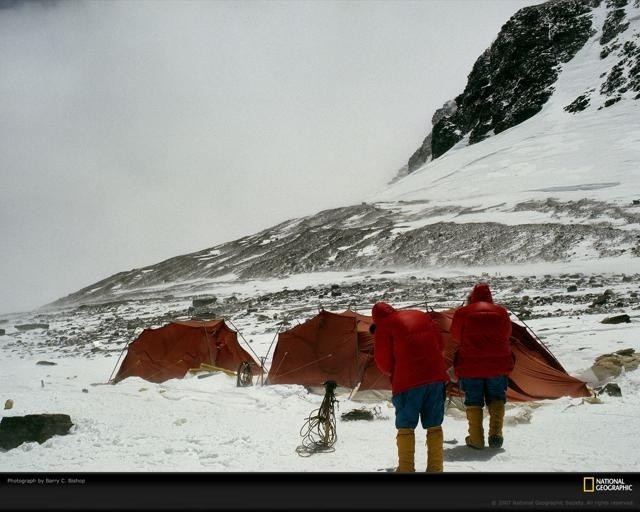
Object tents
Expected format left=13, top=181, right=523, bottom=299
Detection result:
left=265, top=307, right=592, bottom=402
left=109, top=319, right=265, bottom=383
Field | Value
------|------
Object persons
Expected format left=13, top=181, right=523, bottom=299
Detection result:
left=445, top=282, right=516, bottom=449
left=372, top=302, right=450, bottom=472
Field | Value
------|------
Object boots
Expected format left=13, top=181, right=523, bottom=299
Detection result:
left=488, top=401, right=505, bottom=449
left=427, top=426, right=443, bottom=473
left=396, top=429, right=416, bottom=473
left=465, top=406, right=484, bottom=450
